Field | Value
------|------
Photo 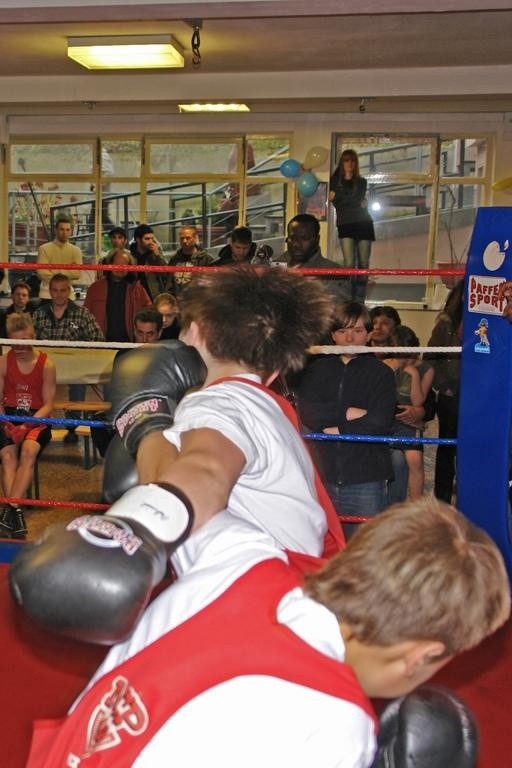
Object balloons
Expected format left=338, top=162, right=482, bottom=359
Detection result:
left=280, top=159, right=301, bottom=177
left=297, top=173, right=317, bottom=196
left=302, top=146, right=328, bottom=170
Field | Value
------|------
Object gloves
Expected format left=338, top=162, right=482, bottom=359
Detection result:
left=6, top=479, right=195, bottom=644
left=107, top=338, right=208, bottom=454
left=372, top=683, right=480, bottom=766
left=100, top=435, right=139, bottom=502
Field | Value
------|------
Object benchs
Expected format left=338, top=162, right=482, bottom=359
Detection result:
left=52, top=400, right=112, bottom=421
left=75, top=425, right=99, bottom=470
left=26, top=429, right=69, bottom=509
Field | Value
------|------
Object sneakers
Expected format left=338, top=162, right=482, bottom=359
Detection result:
left=64, top=434, right=79, bottom=444
left=11, top=509, right=28, bottom=536
left=0, top=503, right=16, bottom=537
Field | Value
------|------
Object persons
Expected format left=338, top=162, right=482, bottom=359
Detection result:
left=1, top=210, right=512, bottom=545
left=89, top=143, right=116, bottom=233
left=220, top=184, right=263, bottom=245
left=328, top=149, right=376, bottom=303
left=25, top=339, right=512, bottom=768
left=8, top=260, right=348, bottom=646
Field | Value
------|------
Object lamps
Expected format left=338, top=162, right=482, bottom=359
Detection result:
left=178, top=102, right=250, bottom=112
left=65, top=35, right=185, bottom=71
